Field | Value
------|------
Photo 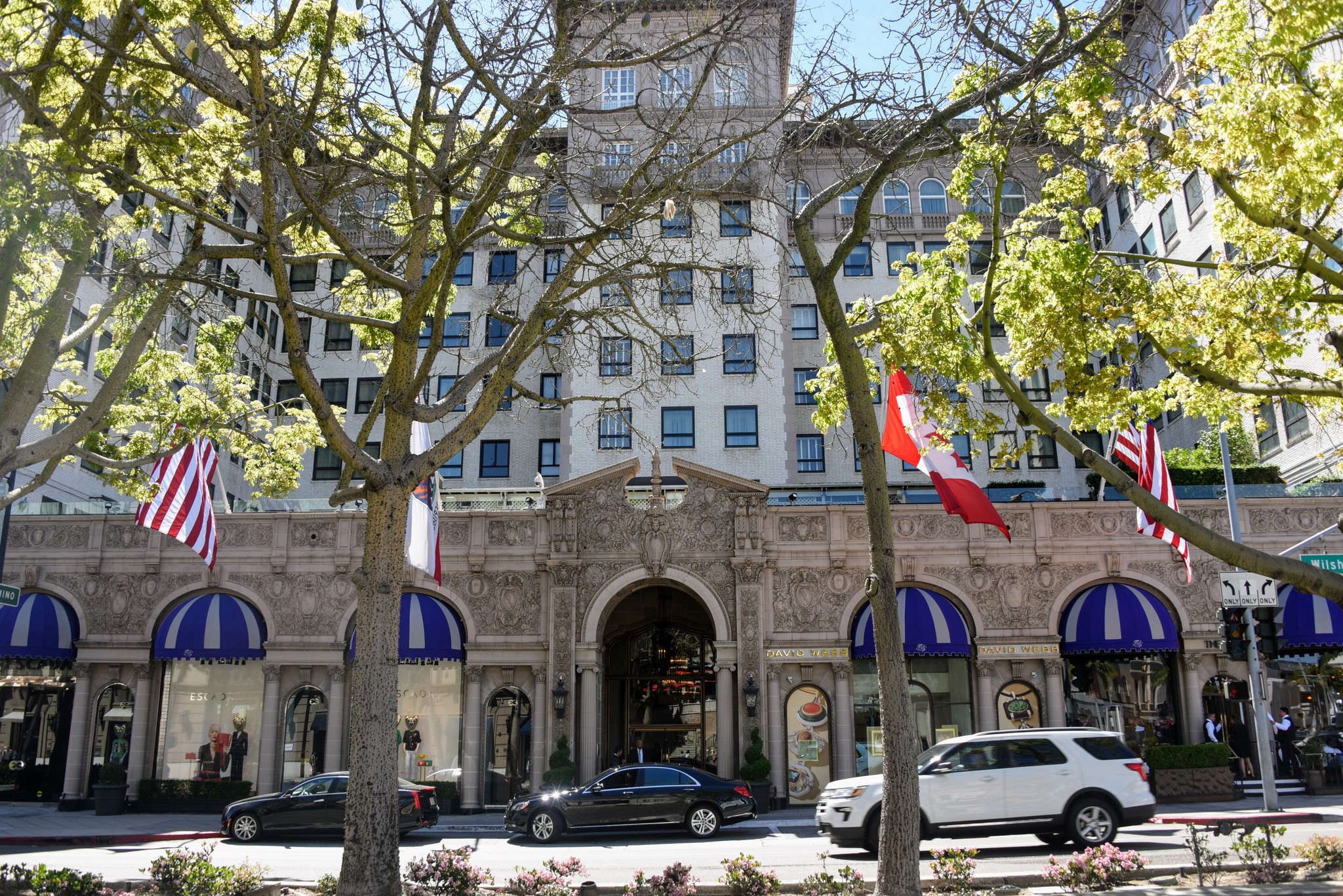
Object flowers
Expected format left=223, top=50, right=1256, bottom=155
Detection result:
left=98, top=762, right=127, bottom=786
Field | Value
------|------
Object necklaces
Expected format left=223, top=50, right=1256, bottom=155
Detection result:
left=210, top=745, right=216, bottom=751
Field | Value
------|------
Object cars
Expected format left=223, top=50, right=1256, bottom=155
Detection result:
left=220, top=771, right=439, bottom=842
left=503, top=763, right=757, bottom=844
left=456, top=769, right=527, bottom=799
left=1293, top=731, right=1343, bottom=768
left=854, top=741, right=883, bottom=775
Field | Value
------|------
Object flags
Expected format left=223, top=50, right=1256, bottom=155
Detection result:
left=881, top=357, right=1013, bottom=544
left=133, top=375, right=219, bottom=572
left=405, top=372, right=442, bottom=589
left=1113, top=365, right=1194, bottom=584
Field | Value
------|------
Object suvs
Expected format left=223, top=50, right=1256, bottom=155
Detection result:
left=814, top=727, right=1157, bottom=857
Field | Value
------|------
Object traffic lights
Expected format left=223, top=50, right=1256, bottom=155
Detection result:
left=1304, top=694, right=1313, bottom=701
left=1224, top=679, right=1251, bottom=701
left=1329, top=672, right=1337, bottom=681
left=1221, top=606, right=1252, bottom=657
left=1251, top=606, right=1279, bottom=658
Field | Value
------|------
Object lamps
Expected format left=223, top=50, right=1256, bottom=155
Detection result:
left=105, top=502, right=112, bottom=510
left=525, top=497, right=534, bottom=506
left=355, top=499, right=363, bottom=508
left=743, top=677, right=759, bottom=717
left=788, top=493, right=797, bottom=501
left=19, top=497, right=28, bottom=512
left=1009, top=491, right=1049, bottom=502
left=46, top=716, right=54, bottom=730
left=551, top=675, right=568, bottom=719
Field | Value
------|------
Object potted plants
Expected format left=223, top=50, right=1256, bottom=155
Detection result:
left=740, top=726, right=771, bottom=814
left=410, top=780, right=457, bottom=815
left=1299, top=737, right=1326, bottom=789
left=138, top=779, right=254, bottom=814
left=539, top=733, right=577, bottom=791
left=1145, top=743, right=1233, bottom=797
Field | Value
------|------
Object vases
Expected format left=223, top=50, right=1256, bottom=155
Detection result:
left=91, top=784, right=129, bottom=815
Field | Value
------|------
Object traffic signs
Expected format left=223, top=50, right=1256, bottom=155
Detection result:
left=1218, top=572, right=1279, bottom=608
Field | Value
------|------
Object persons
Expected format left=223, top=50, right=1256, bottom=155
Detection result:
left=396, top=730, right=422, bottom=782
left=195, top=723, right=230, bottom=780
left=1203, top=712, right=1255, bottom=780
left=1270, top=707, right=1300, bottom=779
left=229, top=730, right=248, bottom=781
left=627, top=739, right=654, bottom=764
left=609, top=746, right=632, bottom=788
left=309, top=749, right=318, bottom=777
left=0, top=746, right=17, bottom=782
left=110, top=738, right=129, bottom=770
left=680, top=750, right=690, bottom=758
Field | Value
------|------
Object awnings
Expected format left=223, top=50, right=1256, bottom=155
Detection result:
left=0, top=592, right=467, bottom=663
left=102, top=706, right=133, bottom=721
left=850, top=583, right=1343, bottom=660
left=0, top=711, right=24, bottom=723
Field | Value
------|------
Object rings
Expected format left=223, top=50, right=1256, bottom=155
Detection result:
left=226, top=748, right=226, bottom=749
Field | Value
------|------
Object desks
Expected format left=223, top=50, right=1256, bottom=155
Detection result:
left=1149, top=813, right=1343, bottom=887
